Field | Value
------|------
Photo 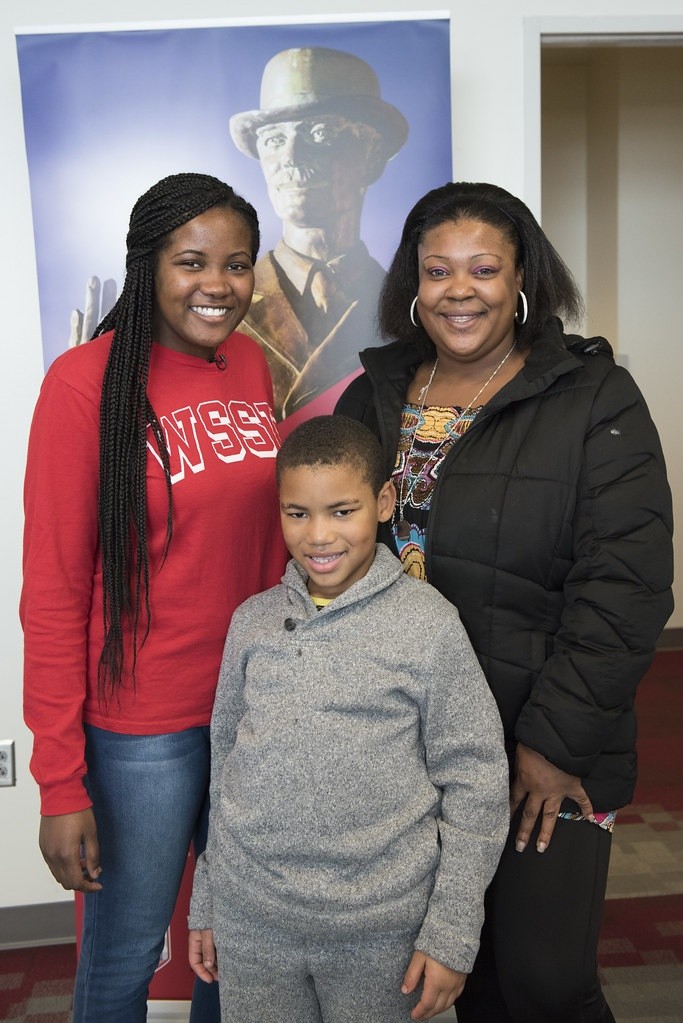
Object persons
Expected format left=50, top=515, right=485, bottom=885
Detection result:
left=18, top=174, right=293, bottom=1023
left=186, top=414, right=509, bottom=1023
left=336, top=183, right=673, bottom=1023
left=69, top=47, right=410, bottom=422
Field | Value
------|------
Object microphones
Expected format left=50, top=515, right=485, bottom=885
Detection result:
left=219, top=353, right=226, bottom=363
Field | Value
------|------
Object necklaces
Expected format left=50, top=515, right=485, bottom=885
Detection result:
left=395, top=340, right=517, bottom=539
